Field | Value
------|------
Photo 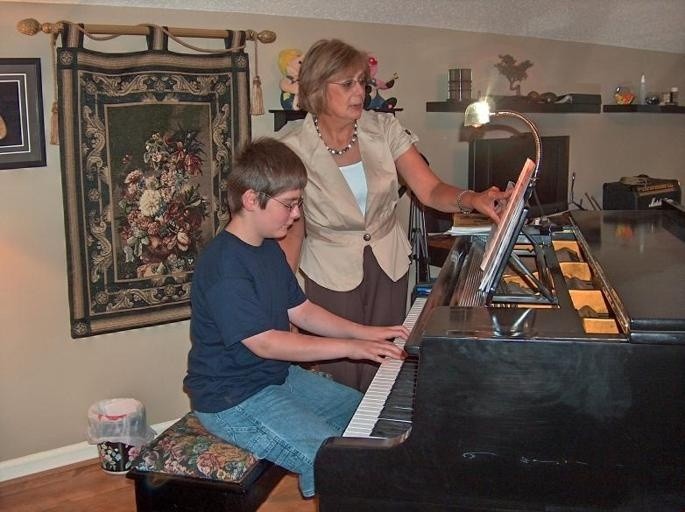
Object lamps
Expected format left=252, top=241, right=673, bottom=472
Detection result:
left=463, top=101, right=543, bottom=234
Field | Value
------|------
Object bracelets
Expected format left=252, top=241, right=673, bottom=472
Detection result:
left=455, top=189, right=476, bottom=216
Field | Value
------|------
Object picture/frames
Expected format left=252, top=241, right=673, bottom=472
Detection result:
left=0, top=58, right=46, bottom=169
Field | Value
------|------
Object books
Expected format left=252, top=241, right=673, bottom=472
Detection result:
left=449, top=212, right=493, bottom=236
left=475, top=179, right=525, bottom=292
left=635, top=185, right=679, bottom=198
left=480, top=156, right=537, bottom=271
left=554, top=93, right=602, bottom=105
left=604, top=175, right=679, bottom=193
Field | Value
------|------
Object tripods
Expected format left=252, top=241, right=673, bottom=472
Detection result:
left=408, top=194, right=430, bottom=283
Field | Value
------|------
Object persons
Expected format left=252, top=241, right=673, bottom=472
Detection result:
left=277, top=46, right=305, bottom=111
left=183, top=134, right=411, bottom=512
left=362, top=54, right=399, bottom=110
left=277, top=35, right=516, bottom=398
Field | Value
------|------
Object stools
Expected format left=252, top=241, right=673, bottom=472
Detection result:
left=126, top=369, right=332, bottom=511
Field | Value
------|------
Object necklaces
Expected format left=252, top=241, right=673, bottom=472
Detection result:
left=313, top=114, right=358, bottom=156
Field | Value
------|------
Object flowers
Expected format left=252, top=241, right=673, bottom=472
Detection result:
left=114, top=119, right=211, bottom=279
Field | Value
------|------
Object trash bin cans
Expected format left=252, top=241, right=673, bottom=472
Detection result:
left=88, top=398, right=145, bottom=475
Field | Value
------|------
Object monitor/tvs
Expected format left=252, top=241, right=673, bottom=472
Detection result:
left=470, top=135, right=572, bottom=216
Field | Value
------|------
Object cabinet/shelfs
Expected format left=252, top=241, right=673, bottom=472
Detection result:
left=604, top=175, right=681, bottom=210
left=424, top=98, right=684, bottom=113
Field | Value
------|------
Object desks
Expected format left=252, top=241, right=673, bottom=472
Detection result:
left=417, top=216, right=574, bottom=286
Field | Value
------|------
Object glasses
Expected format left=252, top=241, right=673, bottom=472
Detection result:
left=262, top=191, right=303, bottom=212
left=327, top=76, right=370, bottom=87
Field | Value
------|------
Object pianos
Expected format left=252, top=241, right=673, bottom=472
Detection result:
left=313, top=199, right=683, bottom=507
